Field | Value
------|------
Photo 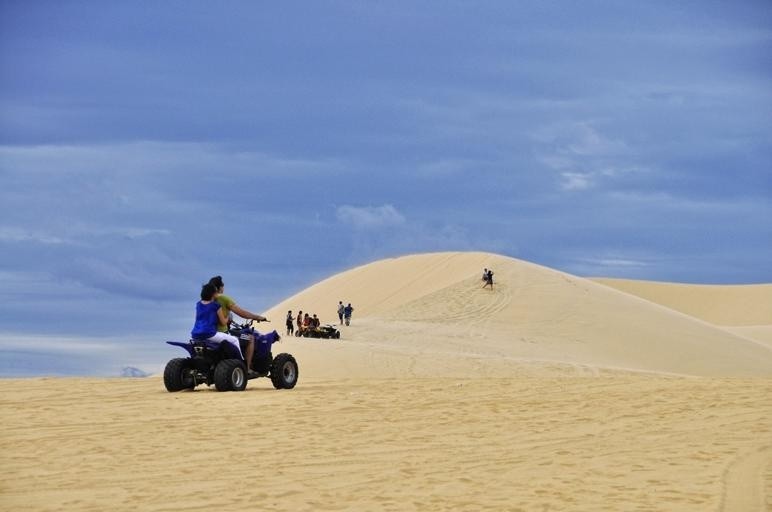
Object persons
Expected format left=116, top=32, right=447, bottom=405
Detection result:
left=286, top=310, right=320, bottom=336
left=337, top=301, right=354, bottom=326
left=482, top=268, right=494, bottom=289
left=190, top=275, right=267, bottom=376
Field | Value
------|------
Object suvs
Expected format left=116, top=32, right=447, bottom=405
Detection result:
left=297, top=324, right=342, bottom=338
left=164, top=318, right=298, bottom=395
